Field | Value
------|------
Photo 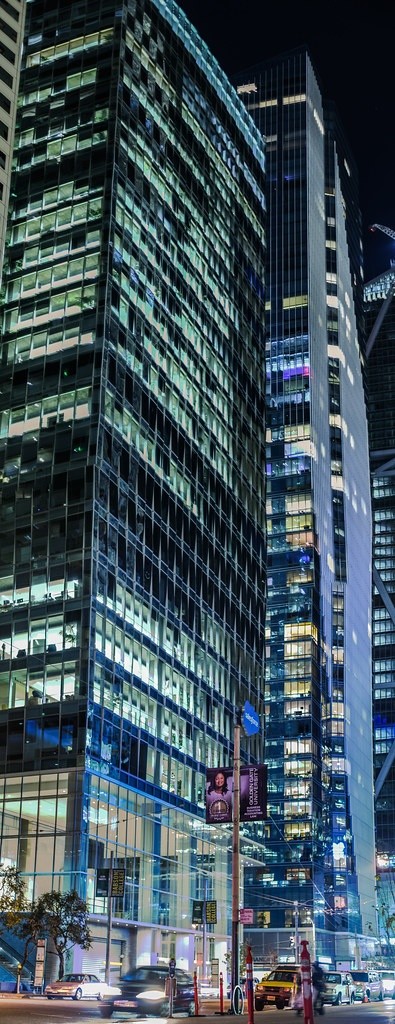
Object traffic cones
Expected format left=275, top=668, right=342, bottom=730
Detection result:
left=361, top=991, right=369, bottom=1003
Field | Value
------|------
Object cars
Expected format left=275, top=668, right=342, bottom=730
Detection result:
left=227, top=976, right=258, bottom=998
left=254, top=961, right=395, bottom=1010
left=44, top=973, right=107, bottom=1001
left=100, top=965, right=203, bottom=1018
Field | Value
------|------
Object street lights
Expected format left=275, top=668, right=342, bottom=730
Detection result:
left=294, top=898, right=325, bottom=965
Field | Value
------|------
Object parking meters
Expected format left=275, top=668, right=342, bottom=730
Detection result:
left=167, top=958, right=177, bottom=1019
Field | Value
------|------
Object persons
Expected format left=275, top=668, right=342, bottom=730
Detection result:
left=207, top=771, right=233, bottom=806
